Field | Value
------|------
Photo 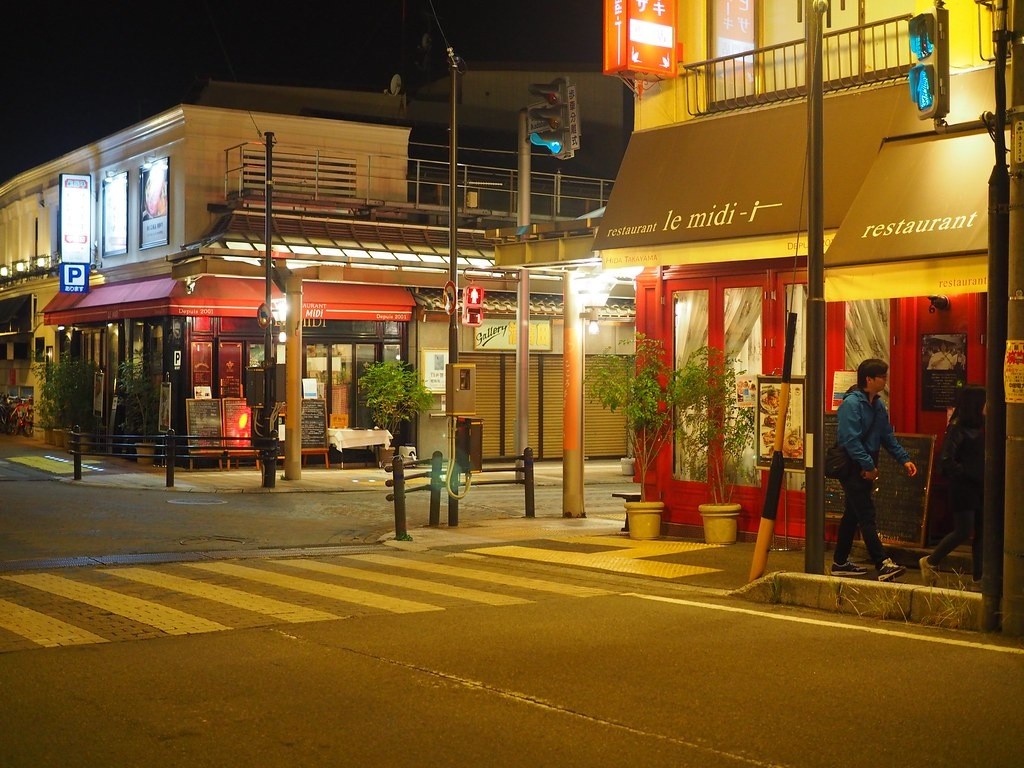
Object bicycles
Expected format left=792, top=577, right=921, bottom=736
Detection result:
left=1, top=393, right=36, bottom=437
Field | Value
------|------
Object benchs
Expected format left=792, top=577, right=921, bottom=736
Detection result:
left=612, top=493, right=642, bottom=532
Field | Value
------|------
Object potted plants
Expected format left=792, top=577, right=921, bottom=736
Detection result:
left=359, top=357, right=435, bottom=465
left=670, top=344, right=760, bottom=546
left=114, top=348, right=161, bottom=464
left=29, top=343, right=98, bottom=454
left=584, top=327, right=684, bottom=540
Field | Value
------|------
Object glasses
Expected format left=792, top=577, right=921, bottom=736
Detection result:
left=874, top=375, right=886, bottom=379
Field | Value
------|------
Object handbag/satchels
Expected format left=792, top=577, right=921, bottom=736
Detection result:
left=825, top=447, right=849, bottom=479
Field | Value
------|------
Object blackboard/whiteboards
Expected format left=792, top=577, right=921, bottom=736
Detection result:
left=222, top=397, right=261, bottom=453
left=823, top=412, right=847, bottom=517
left=300, top=398, right=330, bottom=454
left=186, top=398, right=223, bottom=453
left=919, top=366, right=965, bottom=411
left=870, top=432, right=936, bottom=549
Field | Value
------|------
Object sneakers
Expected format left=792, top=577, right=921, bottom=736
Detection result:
left=971, top=578, right=984, bottom=592
left=877, top=558, right=906, bottom=581
left=919, top=556, right=937, bottom=587
left=831, top=561, right=869, bottom=576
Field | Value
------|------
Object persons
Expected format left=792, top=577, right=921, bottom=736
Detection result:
left=830, top=359, right=916, bottom=582
left=919, top=385, right=982, bottom=591
left=922, top=343, right=965, bottom=371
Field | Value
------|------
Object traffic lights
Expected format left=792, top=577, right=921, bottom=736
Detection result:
left=464, top=287, right=483, bottom=327
left=909, top=8, right=949, bottom=122
left=529, top=76, right=575, bottom=161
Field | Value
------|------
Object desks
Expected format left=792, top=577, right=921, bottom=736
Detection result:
left=327, top=428, right=393, bottom=471
